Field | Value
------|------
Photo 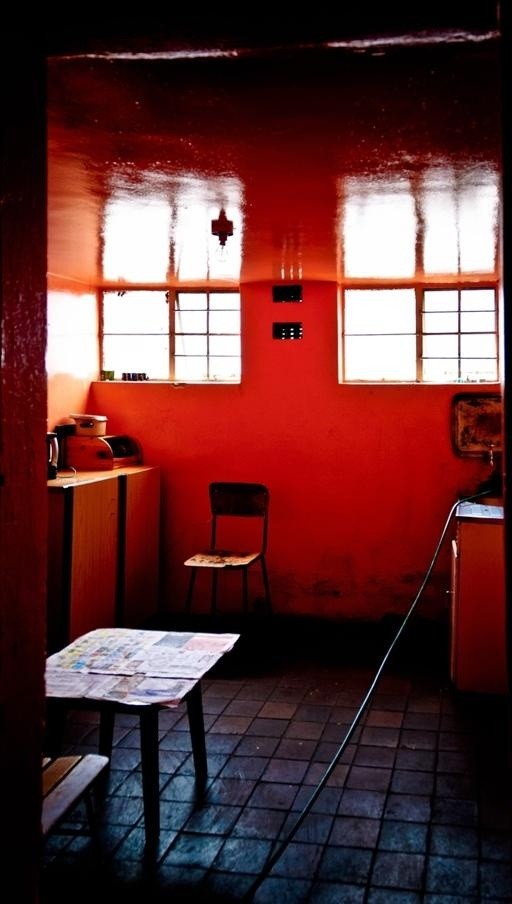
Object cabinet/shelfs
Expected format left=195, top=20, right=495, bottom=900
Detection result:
left=47, top=464, right=161, bottom=642
left=450, top=496, right=505, bottom=693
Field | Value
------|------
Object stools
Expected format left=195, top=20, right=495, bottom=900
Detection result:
left=41, top=755, right=112, bottom=866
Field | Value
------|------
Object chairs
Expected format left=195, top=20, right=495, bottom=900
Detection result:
left=185, top=482, right=271, bottom=615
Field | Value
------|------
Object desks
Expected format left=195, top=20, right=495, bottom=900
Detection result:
left=44, top=628, right=240, bottom=858
